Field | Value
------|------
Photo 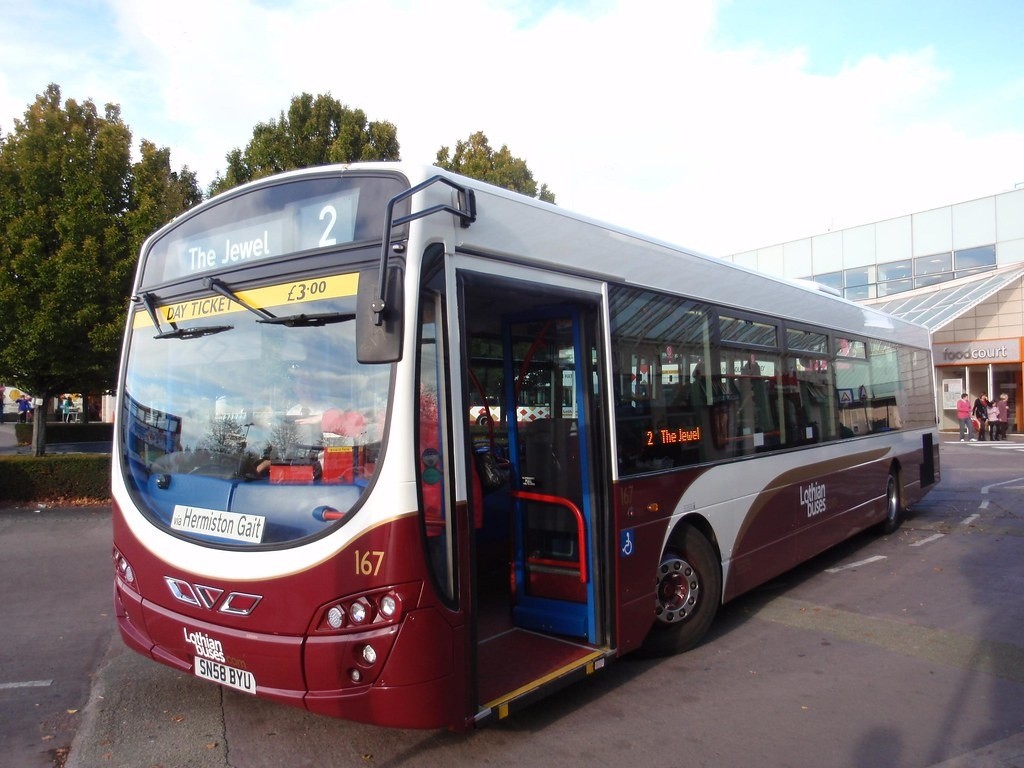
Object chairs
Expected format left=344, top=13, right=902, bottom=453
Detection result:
left=195, top=371, right=901, bottom=562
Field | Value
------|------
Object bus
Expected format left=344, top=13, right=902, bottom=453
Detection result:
left=109, top=157, right=942, bottom=732
left=470, top=353, right=578, bottom=426
left=625, top=277, right=853, bottom=407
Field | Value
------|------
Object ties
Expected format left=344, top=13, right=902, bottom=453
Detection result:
left=301, top=407, right=311, bottom=415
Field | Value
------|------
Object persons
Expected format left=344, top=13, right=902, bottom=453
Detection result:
left=957, top=393, right=977, bottom=442
left=64, top=397, right=74, bottom=423
left=15, top=395, right=34, bottom=423
left=293, top=374, right=442, bottom=537
left=987, top=400, right=1000, bottom=441
left=996, top=394, right=1009, bottom=440
left=972, top=392, right=992, bottom=441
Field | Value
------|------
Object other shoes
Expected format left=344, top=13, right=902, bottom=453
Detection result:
left=1002, top=437, right=1007, bottom=440
left=969, top=438, right=978, bottom=442
left=961, top=438, right=965, bottom=442
left=990, top=438, right=995, bottom=441
left=994, top=436, right=1001, bottom=441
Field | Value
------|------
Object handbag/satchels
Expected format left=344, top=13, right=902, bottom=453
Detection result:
left=971, top=415, right=979, bottom=428
left=982, top=412, right=988, bottom=420
